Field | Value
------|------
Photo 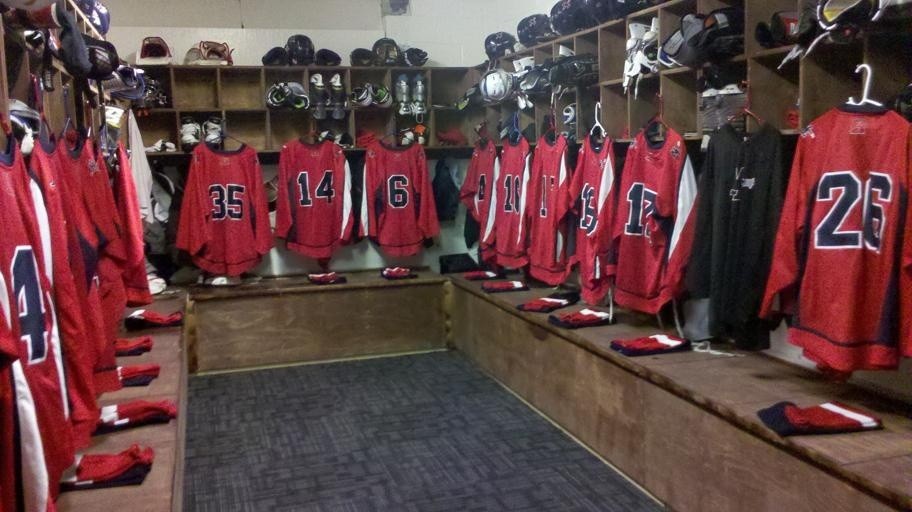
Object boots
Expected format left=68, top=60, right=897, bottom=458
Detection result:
left=622, top=38, right=641, bottom=100
left=627, top=40, right=659, bottom=77
left=265, top=82, right=428, bottom=124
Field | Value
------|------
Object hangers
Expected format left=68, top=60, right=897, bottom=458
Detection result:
left=718, top=77, right=774, bottom=143
left=631, top=91, right=682, bottom=146
left=539, top=100, right=572, bottom=148
left=473, top=107, right=533, bottom=150
left=298, top=111, right=324, bottom=145
left=834, top=63, right=887, bottom=118
left=0, top=65, right=120, bottom=161
left=208, top=120, right=247, bottom=149
left=378, top=110, right=412, bottom=146
left=578, top=99, right=615, bottom=149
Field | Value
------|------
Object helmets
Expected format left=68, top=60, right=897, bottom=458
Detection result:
left=484, top=0, right=653, bottom=62
left=184, top=40, right=236, bottom=66
left=90, top=1, right=111, bottom=37
left=139, top=35, right=172, bottom=60
left=284, top=34, right=314, bottom=65
left=373, top=38, right=402, bottom=65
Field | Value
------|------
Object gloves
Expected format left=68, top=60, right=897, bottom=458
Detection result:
left=402, top=47, right=428, bottom=66
left=350, top=48, right=374, bottom=66
left=262, top=47, right=287, bottom=66
left=315, top=49, right=341, bottom=66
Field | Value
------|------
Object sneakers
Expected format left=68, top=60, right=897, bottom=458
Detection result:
left=143, top=138, right=176, bottom=152
left=181, top=115, right=201, bottom=151
left=202, top=116, right=221, bottom=146
left=754, top=0, right=912, bottom=71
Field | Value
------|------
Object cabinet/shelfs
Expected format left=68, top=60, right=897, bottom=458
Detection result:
left=482, top=1, right=910, bottom=146
left=192, top=269, right=450, bottom=376
left=1, top=1, right=105, bottom=164
left=451, top=263, right=912, bottom=509
left=42, top=279, right=191, bottom=512
left=119, top=60, right=481, bottom=158
